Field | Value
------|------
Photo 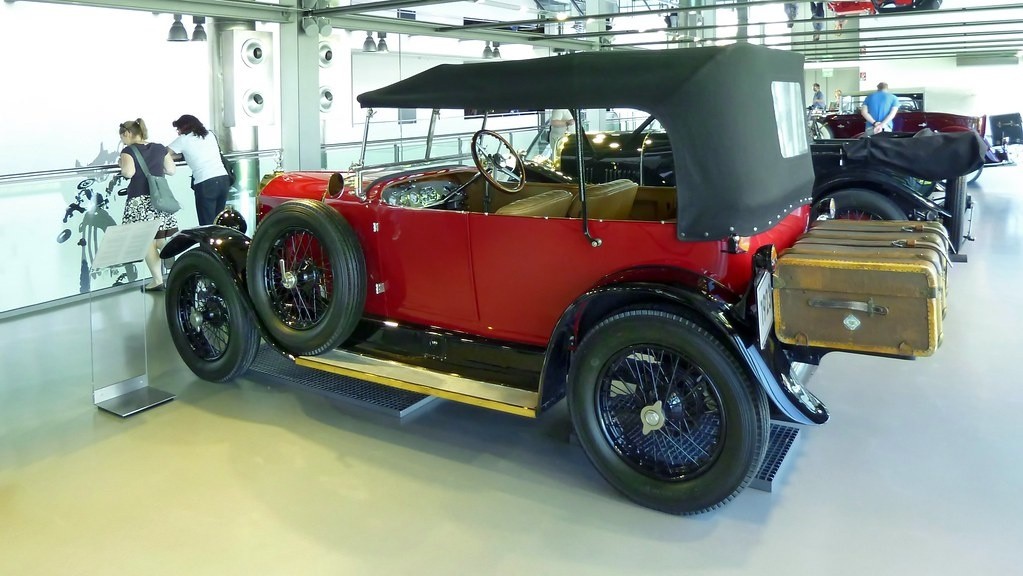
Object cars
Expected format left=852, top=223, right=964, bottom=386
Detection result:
left=155, top=40, right=989, bottom=521
left=827, top=0, right=943, bottom=14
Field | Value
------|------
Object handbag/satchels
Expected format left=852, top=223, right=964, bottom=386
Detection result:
left=191, top=153, right=235, bottom=191
left=147, top=174, right=181, bottom=215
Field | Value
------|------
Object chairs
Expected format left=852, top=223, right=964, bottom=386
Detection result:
left=495, top=188, right=573, bottom=218
left=567, top=179, right=637, bottom=219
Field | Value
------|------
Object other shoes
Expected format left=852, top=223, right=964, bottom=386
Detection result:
left=164, top=256, right=175, bottom=269
left=141, top=282, right=163, bottom=292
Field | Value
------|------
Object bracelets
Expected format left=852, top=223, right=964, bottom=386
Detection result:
left=873, top=121, right=876, bottom=124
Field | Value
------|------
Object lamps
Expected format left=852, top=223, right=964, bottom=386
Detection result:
left=315, top=17, right=332, bottom=37
left=376, top=31, right=389, bottom=54
left=166, top=14, right=190, bottom=41
left=191, top=16, right=208, bottom=41
left=482, top=41, right=494, bottom=60
left=363, top=31, right=377, bottom=53
left=493, top=42, right=501, bottom=59
left=302, top=16, right=319, bottom=37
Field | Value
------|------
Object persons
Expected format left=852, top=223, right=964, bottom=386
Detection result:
left=165, top=115, right=231, bottom=225
left=813, top=83, right=825, bottom=107
left=783, top=1, right=798, bottom=28
left=835, top=14, right=848, bottom=35
left=810, top=1, right=824, bottom=40
left=861, top=82, right=899, bottom=132
left=834, top=89, right=842, bottom=105
left=118, top=118, right=177, bottom=292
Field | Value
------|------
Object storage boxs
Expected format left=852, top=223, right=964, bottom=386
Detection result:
left=771, top=218, right=952, bottom=357
